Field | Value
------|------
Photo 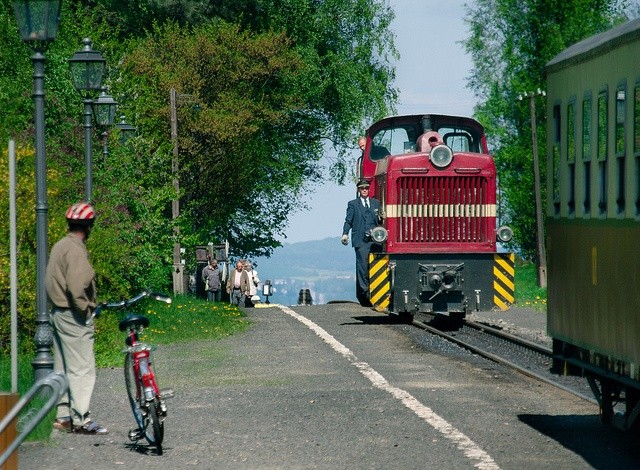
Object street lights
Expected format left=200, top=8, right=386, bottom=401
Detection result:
left=515, top=87, right=546, bottom=289
left=12, top=0, right=62, bottom=395
left=92, top=85, right=120, bottom=174
left=67, top=36, right=106, bottom=201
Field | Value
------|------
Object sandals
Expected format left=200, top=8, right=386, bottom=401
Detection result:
left=50, top=416, right=74, bottom=432
left=75, top=421, right=108, bottom=435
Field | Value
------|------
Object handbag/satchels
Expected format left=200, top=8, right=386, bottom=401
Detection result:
left=252, top=269, right=257, bottom=287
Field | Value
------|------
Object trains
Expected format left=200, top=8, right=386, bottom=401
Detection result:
left=545, top=13, right=640, bottom=430
left=356, top=114, right=515, bottom=318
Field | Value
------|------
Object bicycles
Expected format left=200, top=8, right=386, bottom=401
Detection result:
left=85, top=289, right=175, bottom=455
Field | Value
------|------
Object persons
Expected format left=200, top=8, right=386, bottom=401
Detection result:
left=202, top=259, right=223, bottom=302
left=243, top=263, right=259, bottom=302
left=44, top=201, right=111, bottom=434
left=341, top=179, right=383, bottom=307
left=226, top=261, right=249, bottom=308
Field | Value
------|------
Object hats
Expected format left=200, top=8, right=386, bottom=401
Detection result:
left=356, top=181, right=370, bottom=189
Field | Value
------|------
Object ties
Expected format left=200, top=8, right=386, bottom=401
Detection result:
left=364, top=200, right=369, bottom=208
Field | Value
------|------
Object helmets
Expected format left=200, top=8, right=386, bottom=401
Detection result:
left=65, top=203, right=95, bottom=224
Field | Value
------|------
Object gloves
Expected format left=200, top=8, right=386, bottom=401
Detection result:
left=341, top=234, right=348, bottom=245
